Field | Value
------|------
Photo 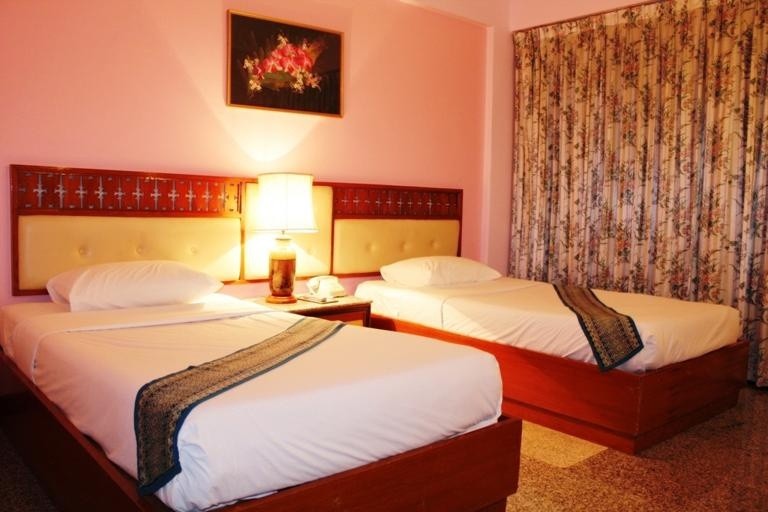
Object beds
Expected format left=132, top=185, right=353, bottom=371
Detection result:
left=1, top=291, right=522, bottom=511
left=355, top=274, right=750, bottom=456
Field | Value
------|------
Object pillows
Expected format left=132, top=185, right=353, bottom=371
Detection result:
left=379, top=255, right=503, bottom=287
left=47, top=260, right=225, bottom=313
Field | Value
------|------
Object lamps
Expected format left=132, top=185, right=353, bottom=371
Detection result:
left=253, top=172, right=320, bottom=305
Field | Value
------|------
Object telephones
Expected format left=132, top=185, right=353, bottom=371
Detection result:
left=307, top=276, right=346, bottom=297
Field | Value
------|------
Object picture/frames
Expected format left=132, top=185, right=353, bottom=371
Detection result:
left=224, top=8, right=346, bottom=121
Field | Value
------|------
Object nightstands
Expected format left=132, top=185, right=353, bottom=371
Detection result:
left=254, top=291, right=372, bottom=328
